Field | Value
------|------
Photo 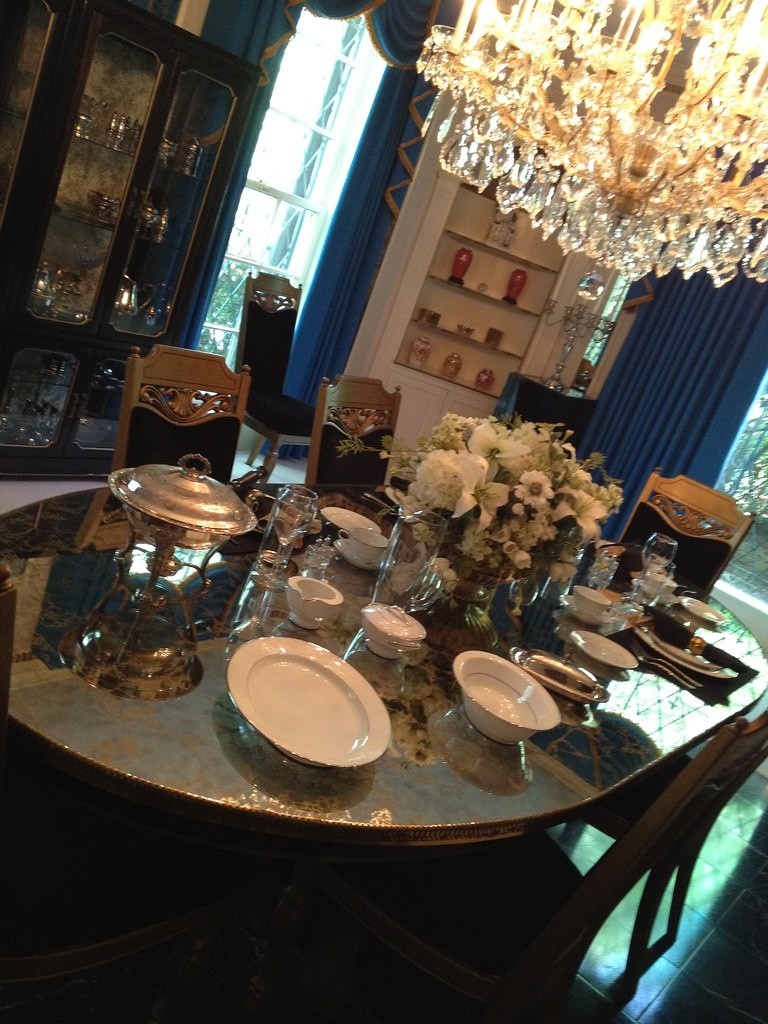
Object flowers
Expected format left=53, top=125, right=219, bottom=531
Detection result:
left=333, top=389, right=627, bottom=571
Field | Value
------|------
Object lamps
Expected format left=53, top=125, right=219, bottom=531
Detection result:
left=414, top=0, right=768, bottom=290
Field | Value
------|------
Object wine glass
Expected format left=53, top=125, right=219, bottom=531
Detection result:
left=249, top=484, right=318, bottom=591
left=619, top=533, right=679, bottom=614
left=632, top=577, right=679, bottom=606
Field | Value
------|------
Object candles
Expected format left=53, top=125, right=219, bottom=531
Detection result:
left=548, top=249, right=620, bottom=318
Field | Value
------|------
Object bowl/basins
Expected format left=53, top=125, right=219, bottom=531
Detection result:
left=453, top=651, right=561, bottom=747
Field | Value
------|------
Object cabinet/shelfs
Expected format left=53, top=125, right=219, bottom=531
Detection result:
left=366, top=360, right=495, bottom=506
left=0, top=0, right=264, bottom=481
left=365, top=175, right=595, bottom=481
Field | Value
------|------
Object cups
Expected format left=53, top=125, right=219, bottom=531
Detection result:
left=573, top=585, right=618, bottom=621
left=286, top=575, right=343, bottom=630
left=336, top=524, right=386, bottom=562
left=586, top=553, right=620, bottom=591
left=371, top=510, right=449, bottom=613
left=259, top=486, right=307, bottom=569
left=631, top=555, right=677, bottom=605
left=649, top=574, right=678, bottom=596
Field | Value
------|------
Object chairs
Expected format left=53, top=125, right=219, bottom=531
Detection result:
left=238, top=702, right=768, bottom=1024
left=577, top=739, right=768, bottom=1001
left=0, top=563, right=247, bottom=1024
left=109, top=340, right=253, bottom=492
left=617, top=468, right=759, bottom=591
left=232, top=270, right=316, bottom=484
left=305, top=371, right=404, bottom=488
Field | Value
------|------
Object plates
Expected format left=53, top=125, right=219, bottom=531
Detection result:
left=386, top=485, right=400, bottom=507
left=510, top=646, right=610, bottom=705
left=570, top=631, right=638, bottom=671
left=331, top=537, right=382, bottom=571
left=559, top=595, right=618, bottom=626
left=633, top=626, right=740, bottom=679
left=320, top=507, right=382, bottom=538
left=648, top=629, right=725, bottom=671
left=678, top=596, right=729, bottom=622
left=225, top=636, right=392, bottom=769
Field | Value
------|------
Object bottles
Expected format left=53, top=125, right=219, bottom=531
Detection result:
left=408, top=246, right=529, bottom=387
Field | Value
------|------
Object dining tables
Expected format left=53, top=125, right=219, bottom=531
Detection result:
left=0, top=489, right=768, bottom=973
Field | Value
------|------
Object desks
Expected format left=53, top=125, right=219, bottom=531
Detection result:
left=489, top=369, right=599, bottom=446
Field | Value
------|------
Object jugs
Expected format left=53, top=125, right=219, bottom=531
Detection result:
left=114, top=272, right=157, bottom=325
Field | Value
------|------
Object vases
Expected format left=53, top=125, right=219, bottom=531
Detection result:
left=449, top=246, right=474, bottom=280
left=503, top=267, right=529, bottom=304
left=408, top=335, right=432, bottom=366
left=443, top=348, right=463, bottom=377
left=405, top=536, right=522, bottom=652
left=476, top=367, right=495, bottom=389
